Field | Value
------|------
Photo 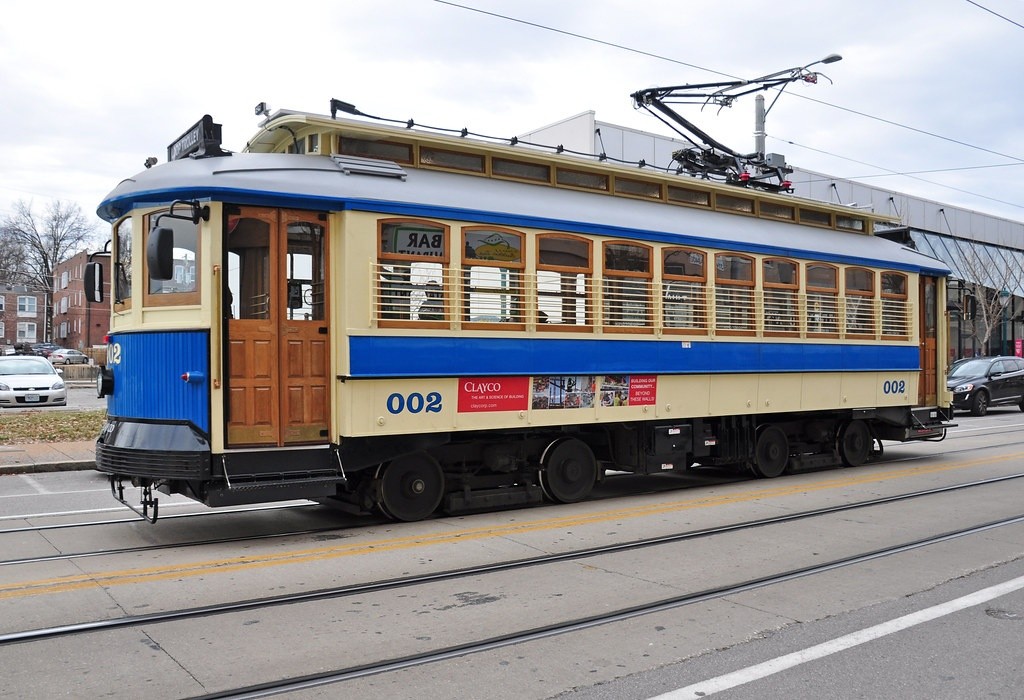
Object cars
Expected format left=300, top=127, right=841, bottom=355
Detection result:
left=0, top=354, right=67, bottom=407
left=947, top=355, right=1024, bottom=416
left=0, top=342, right=88, bottom=364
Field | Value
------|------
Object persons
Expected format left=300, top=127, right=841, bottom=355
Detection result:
left=533, top=376, right=628, bottom=409
left=419, top=281, right=445, bottom=320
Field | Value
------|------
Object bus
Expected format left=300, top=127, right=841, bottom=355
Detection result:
left=84, top=68, right=977, bottom=524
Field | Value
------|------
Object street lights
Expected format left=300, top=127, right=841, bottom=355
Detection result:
left=754, top=53, right=843, bottom=187
